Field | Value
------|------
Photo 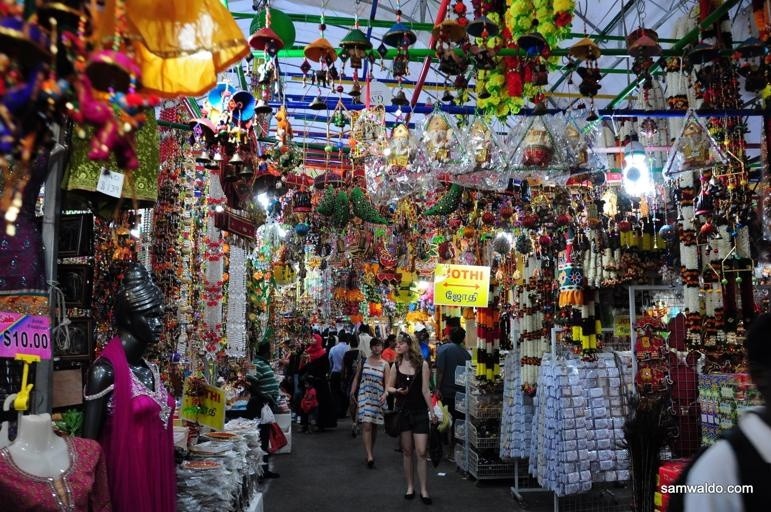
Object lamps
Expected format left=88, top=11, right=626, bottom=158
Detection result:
left=621, top=140, right=651, bottom=196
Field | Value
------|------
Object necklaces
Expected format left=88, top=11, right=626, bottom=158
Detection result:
left=402, top=358, right=413, bottom=381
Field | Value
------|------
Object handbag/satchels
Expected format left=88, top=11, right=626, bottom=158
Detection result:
left=267, top=422, right=287, bottom=453
left=383, top=363, right=421, bottom=438
left=429, top=421, right=443, bottom=467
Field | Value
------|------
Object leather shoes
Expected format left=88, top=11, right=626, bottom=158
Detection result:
left=368, top=457, right=375, bottom=468
left=420, top=490, right=432, bottom=504
left=404, top=489, right=416, bottom=499
left=264, top=471, right=280, bottom=479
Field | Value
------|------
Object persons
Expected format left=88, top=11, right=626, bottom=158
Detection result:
left=387, top=331, right=439, bottom=505
left=77, top=259, right=180, bottom=511
left=300, top=378, right=318, bottom=435
left=328, top=333, right=351, bottom=419
left=302, top=334, right=330, bottom=432
left=279, top=340, right=302, bottom=424
left=341, top=335, right=367, bottom=439
left=350, top=337, right=390, bottom=468
left=414, top=331, right=430, bottom=362
left=666, top=311, right=771, bottom=512
left=357, top=323, right=374, bottom=358
left=436, top=327, right=471, bottom=463
left=246, top=341, right=279, bottom=420
left=381, top=334, right=397, bottom=362
left=0, top=413, right=111, bottom=512
